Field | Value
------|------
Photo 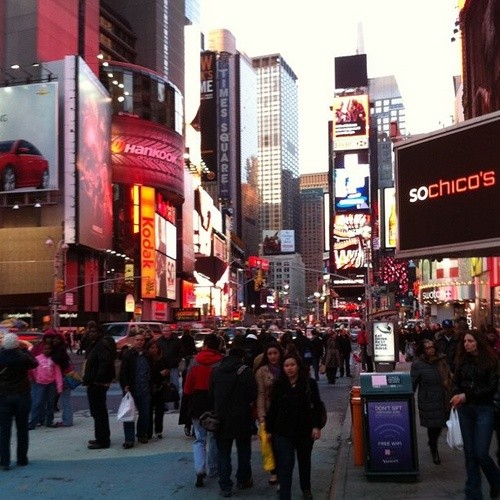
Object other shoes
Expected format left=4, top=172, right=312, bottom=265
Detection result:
left=28, top=421, right=36, bottom=429
left=431, top=453, right=441, bottom=465
left=340, top=374, right=343, bottom=378
left=137, top=436, right=148, bottom=444
left=88, top=442, right=110, bottom=449
left=196, top=473, right=205, bottom=487
left=0, top=466, right=9, bottom=470
left=56, top=422, right=72, bottom=426
left=220, top=487, right=231, bottom=497
left=88, top=439, right=97, bottom=444
left=329, top=381, right=335, bottom=384
left=346, top=374, right=352, bottom=377
left=268, top=475, right=277, bottom=486
left=123, top=440, right=134, bottom=449
left=157, top=433, right=162, bottom=438
left=236, top=478, right=254, bottom=489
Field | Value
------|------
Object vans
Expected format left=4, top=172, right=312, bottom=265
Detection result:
left=101, top=321, right=171, bottom=361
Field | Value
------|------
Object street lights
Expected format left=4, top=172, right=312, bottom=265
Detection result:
left=45, top=234, right=69, bottom=330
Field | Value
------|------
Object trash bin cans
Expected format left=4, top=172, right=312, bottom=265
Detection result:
left=350, top=386, right=364, bottom=465
left=360, top=372, right=421, bottom=483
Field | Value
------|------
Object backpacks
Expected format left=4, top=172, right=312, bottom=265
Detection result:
left=215, top=364, right=248, bottom=413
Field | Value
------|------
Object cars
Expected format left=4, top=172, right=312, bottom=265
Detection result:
left=194, top=332, right=225, bottom=350
left=222, top=328, right=305, bottom=346
left=0, top=139, right=51, bottom=192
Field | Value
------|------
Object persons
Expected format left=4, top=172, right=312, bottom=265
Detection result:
left=397, top=318, right=500, bottom=500
left=0, top=321, right=352, bottom=500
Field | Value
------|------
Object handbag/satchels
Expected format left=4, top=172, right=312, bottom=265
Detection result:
left=258, top=423, right=274, bottom=471
left=118, top=391, right=136, bottom=421
left=308, top=379, right=327, bottom=430
left=319, top=364, right=327, bottom=375
left=445, top=405, right=464, bottom=453
left=64, top=370, right=82, bottom=391
left=160, top=383, right=180, bottom=403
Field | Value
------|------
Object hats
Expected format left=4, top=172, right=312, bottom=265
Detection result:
left=442, top=319, right=452, bottom=327
